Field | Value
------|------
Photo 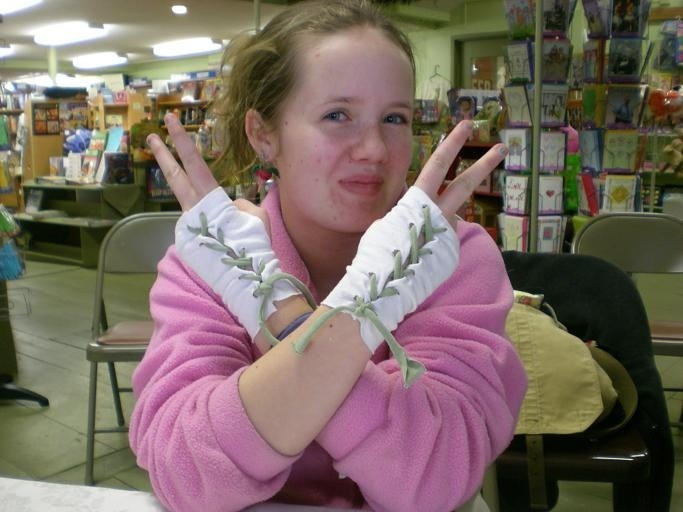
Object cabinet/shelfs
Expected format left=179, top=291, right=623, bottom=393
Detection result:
left=441, top=139, right=505, bottom=199
left=1, top=78, right=240, bottom=268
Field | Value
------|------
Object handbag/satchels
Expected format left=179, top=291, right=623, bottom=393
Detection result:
left=503, top=302, right=637, bottom=510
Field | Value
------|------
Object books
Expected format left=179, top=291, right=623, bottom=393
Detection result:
left=404, top=0, right=682, bottom=261
left=0, top=69, right=278, bottom=283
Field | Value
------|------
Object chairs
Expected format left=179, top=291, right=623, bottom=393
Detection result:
left=84, top=211, right=184, bottom=489
left=483, top=247, right=662, bottom=511
left=569, top=212, right=683, bottom=433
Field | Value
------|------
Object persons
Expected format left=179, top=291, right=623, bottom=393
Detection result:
left=119, top=0, right=528, bottom=510
